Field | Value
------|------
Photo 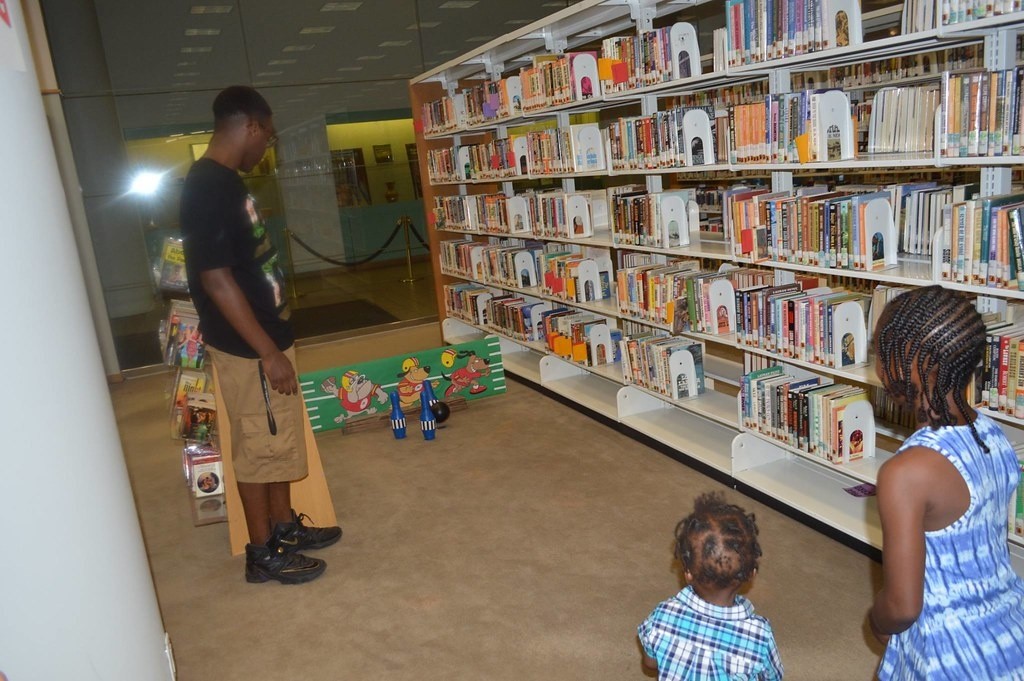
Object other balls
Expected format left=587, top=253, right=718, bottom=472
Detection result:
left=430, top=401, right=451, bottom=424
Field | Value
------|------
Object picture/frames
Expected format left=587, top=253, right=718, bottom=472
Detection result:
left=373, top=144, right=394, bottom=166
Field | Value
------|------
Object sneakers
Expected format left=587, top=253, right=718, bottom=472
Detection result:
left=272, top=508, right=343, bottom=552
left=245, top=534, right=326, bottom=584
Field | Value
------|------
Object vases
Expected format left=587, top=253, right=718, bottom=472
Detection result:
left=384, top=180, right=398, bottom=202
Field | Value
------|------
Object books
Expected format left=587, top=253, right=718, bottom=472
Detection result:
left=423, top=0, right=1024, bottom=543
left=158, top=242, right=222, bottom=499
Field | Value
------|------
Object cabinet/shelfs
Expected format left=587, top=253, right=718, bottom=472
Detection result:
left=147, top=235, right=338, bottom=558
left=409, top=0, right=1023, bottom=581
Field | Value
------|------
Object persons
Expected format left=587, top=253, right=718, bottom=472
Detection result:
left=180, top=85, right=341, bottom=585
left=868, top=284, right=1024, bottom=681
left=637, top=490, right=784, bottom=681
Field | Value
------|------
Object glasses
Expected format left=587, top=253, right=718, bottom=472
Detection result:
left=247, top=122, right=279, bottom=148
